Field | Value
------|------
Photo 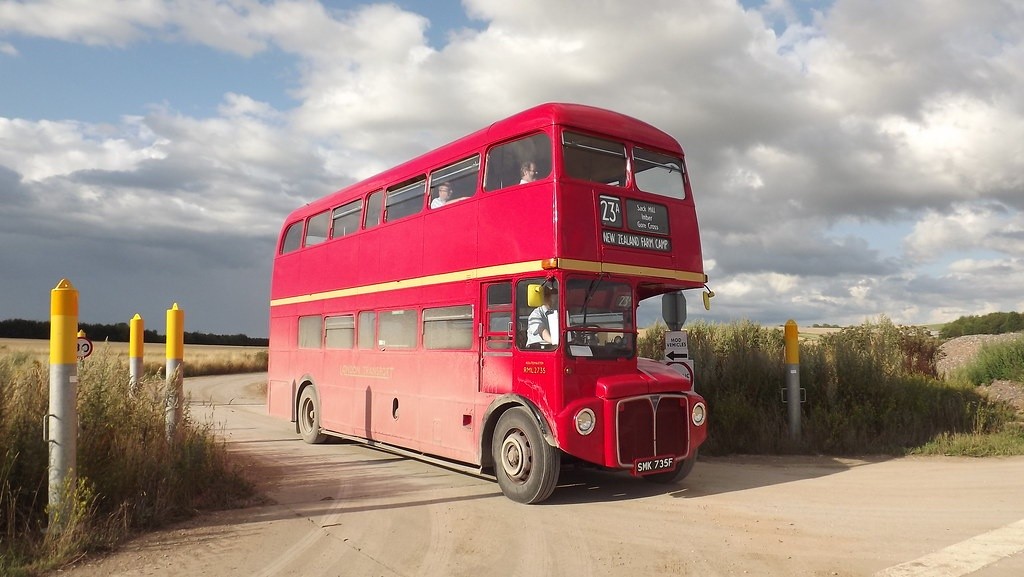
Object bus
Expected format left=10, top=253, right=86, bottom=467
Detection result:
left=264, top=101, right=718, bottom=504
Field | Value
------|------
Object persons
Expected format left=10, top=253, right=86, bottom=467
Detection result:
left=431, top=181, right=471, bottom=210
left=526, top=281, right=559, bottom=349
left=518, top=161, right=540, bottom=185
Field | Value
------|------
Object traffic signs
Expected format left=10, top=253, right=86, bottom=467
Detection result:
left=664, top=331, right=689, bottom=363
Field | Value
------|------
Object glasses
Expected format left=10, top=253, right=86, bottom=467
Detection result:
left=529, top=169, right=541, bottom=175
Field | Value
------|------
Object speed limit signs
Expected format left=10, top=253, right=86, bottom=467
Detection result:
left=77, top=337, right=93, bottom=359
